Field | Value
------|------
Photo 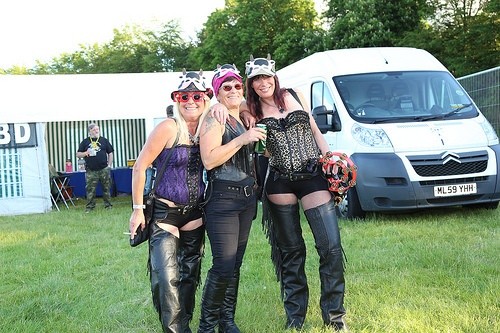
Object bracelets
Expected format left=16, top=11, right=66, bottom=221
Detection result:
left=132, top=205, right=146, bottom=209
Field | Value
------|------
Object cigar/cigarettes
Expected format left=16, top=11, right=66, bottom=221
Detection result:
left=123, top=233, right=137, bottom=235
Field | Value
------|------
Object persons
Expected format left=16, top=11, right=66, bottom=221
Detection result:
left=197, top=63, right=267, bottom=333
left=76, top=124, right=114, bottom=208
left=210, top=54, right=357, bottom=333
left=123, top=71, right=256, bottom=333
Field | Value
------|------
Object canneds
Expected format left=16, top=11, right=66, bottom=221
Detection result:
left=255, top=123, right=267, bottom=154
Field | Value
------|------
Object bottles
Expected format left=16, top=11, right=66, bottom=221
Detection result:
left=78, top=158, right=86, bottom=171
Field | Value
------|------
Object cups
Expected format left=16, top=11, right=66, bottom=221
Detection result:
left=89, top=148, right=96, bottom=156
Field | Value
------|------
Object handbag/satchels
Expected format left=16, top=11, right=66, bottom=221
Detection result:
left=130, top=193, right=155, bottom=247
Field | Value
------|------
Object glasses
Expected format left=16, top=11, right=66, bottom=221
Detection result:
left=174, top=93, right=204, bottom=101
left=219, top=84, right=242, bottom=92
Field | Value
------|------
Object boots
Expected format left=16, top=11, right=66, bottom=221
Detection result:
left=263, top=193, right=309, bottom=332
left=218, top=276, right=241, bottom=333
left=304, top=192, right=347, bottom=332
left=197, top=270, right=233, bottom=333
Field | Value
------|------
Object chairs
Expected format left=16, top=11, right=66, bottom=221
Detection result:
left=335, top=82, right=417, bottom=110
left=49, top=163, right=76, bottom=211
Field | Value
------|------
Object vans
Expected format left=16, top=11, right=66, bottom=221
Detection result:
left=275, top=47, right=500, bottom=220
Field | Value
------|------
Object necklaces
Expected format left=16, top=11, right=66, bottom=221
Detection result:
left=277, top=106, right=285, bottom=113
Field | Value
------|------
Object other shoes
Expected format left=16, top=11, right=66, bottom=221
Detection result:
left=105, top=205, right=112, bottom=211
left=85, top=208, right=93, bottom=214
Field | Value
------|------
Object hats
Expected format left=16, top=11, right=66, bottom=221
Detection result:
left=170, top=67, right=207, bottom=101
left=211, top=63, right=242, bottom=97
left=245, top=52, right=275, bottom=78
left=88, top=124, right=96, bottom=131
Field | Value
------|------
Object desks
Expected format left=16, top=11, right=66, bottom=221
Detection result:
left=60, top=166, right=132, bottom=197
